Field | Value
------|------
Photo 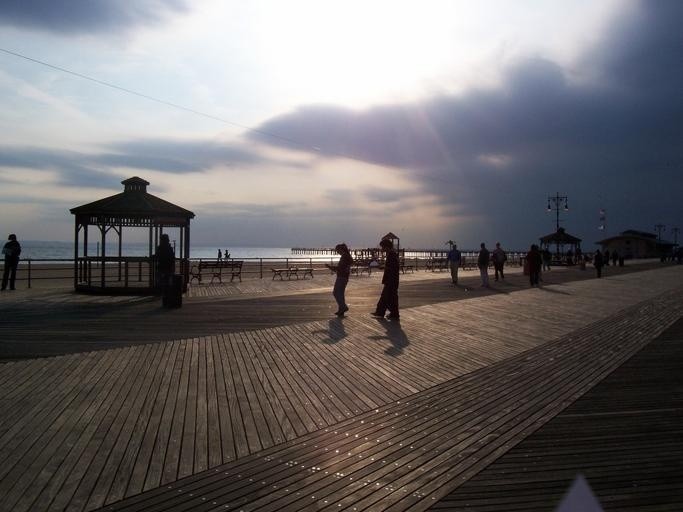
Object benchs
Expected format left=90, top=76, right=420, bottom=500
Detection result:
left=461, top=263, right=478, bottom=271
left=350, top=261, right=372, bottom=275
left=424, top=258, right=450, bottom=274
left=271, top=266, right=314, bottom=280
left=189, top=258, right=244, bottom=285
left=399, top=265, right=414, bottom=274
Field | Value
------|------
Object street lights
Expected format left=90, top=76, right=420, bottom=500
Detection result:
left=655, top=223, right=680, bottom=262
left=547, top=197, right=569, bottom=264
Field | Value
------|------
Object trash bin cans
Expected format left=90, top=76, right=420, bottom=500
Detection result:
left=162, top=274, right=185, bottom=309
left=524, top=257, right=531, bottom=276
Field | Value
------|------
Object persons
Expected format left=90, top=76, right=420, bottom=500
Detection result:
left=224, top=250, right=228, bottom=261
left=217, top=249, right=222, bottom=261
left=444, top=242, right=625, bottom=285
left=328, top=244, right=353, bottom=316
left=370, top=239, right=399, bottom=319
left=0, top=234, right=21, bottom=290
left=155, top=234, right=175, bottom=297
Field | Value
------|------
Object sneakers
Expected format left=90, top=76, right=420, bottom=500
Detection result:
left=387, top=313, right=401, bottom=321
left=370, top=311, right=386, bottom=319
left=334, top=304, right=349, bottom=319
